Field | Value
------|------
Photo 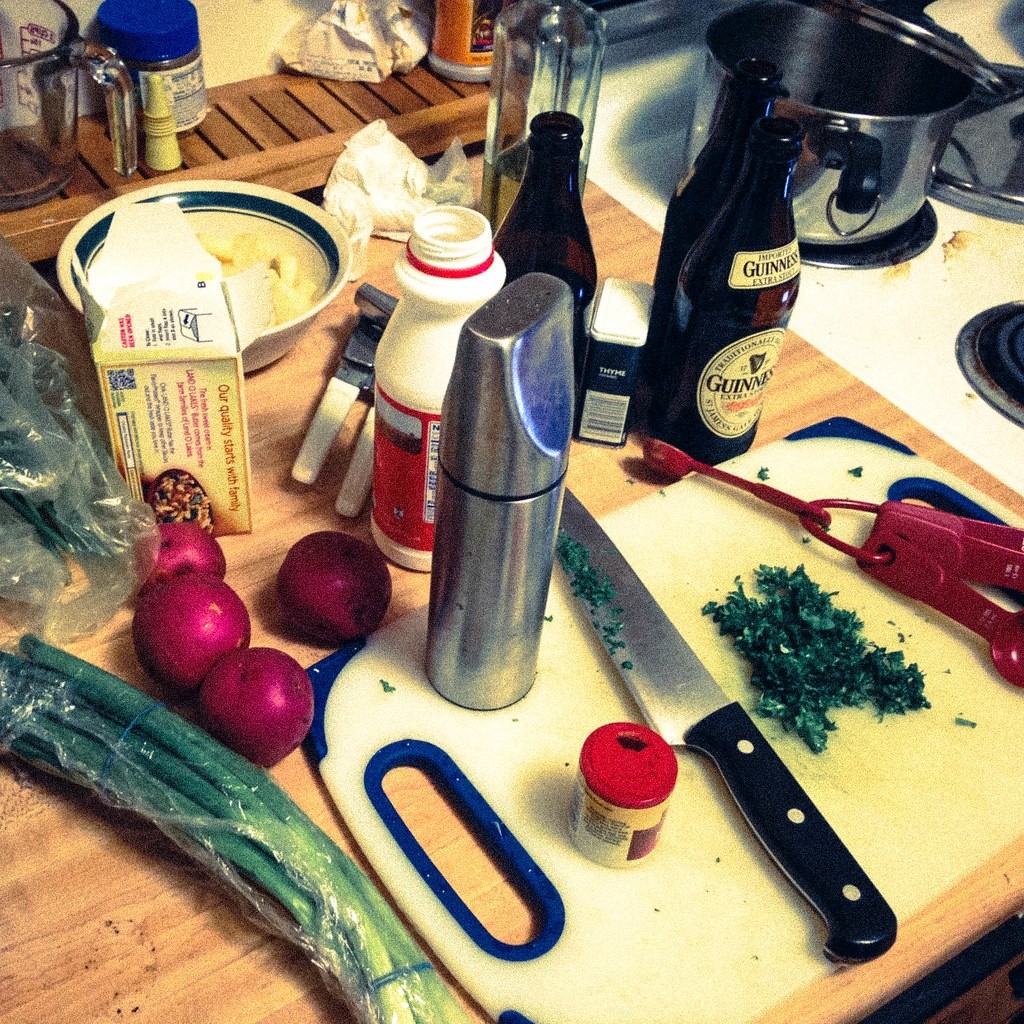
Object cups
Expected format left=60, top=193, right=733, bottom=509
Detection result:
left=0, top=0, right=136, bottom=214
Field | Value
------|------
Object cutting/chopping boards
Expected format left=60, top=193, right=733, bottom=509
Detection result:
left=302, top=417, right=1024, bottom=1023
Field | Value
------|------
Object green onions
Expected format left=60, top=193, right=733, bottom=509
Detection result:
left=0, top=635, right=472, bottom=1024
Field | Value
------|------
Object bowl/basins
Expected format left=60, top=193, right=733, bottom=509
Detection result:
left=55, top=181, right=351, bottom=374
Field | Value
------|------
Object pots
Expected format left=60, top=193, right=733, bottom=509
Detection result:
left=680, top=1, right=1014, bottom=245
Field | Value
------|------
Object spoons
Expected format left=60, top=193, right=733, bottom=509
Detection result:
left=640, top=440, right=833, bottom=528
left=856, top=503, right=1024, bottom=687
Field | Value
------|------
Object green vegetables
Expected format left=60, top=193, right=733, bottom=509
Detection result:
left=1, top=296, right=141, bottom=557
left=557, top=534, right=932, bottom=753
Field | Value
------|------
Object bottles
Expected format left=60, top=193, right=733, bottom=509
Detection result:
left=628, top=59, right=804, bottom=468
left=426, top=1, right=506, bottom=82
left=370, top=205, right=508, bottom=574
left=494, top=111, right=597, bottom=440
left=426, top=273, right=577, bottom=710
left=479, top=0, right=606, bottom=239
left=98, top=1, right=209, bottom=141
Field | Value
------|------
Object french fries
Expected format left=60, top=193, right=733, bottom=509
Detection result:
left=201, top=226, right=315, bottom=325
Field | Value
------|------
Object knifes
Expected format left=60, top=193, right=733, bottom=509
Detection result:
left=555, top=488, right=898, bottom=964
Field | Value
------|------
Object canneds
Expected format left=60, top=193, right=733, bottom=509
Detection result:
left=96, top=0, right=208, bottom=137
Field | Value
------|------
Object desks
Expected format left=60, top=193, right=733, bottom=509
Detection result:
left=0, top=159, right=1023, bottom=1024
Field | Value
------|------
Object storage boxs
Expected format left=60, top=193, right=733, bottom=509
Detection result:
left=63, top=198, right=253, bottom=539
